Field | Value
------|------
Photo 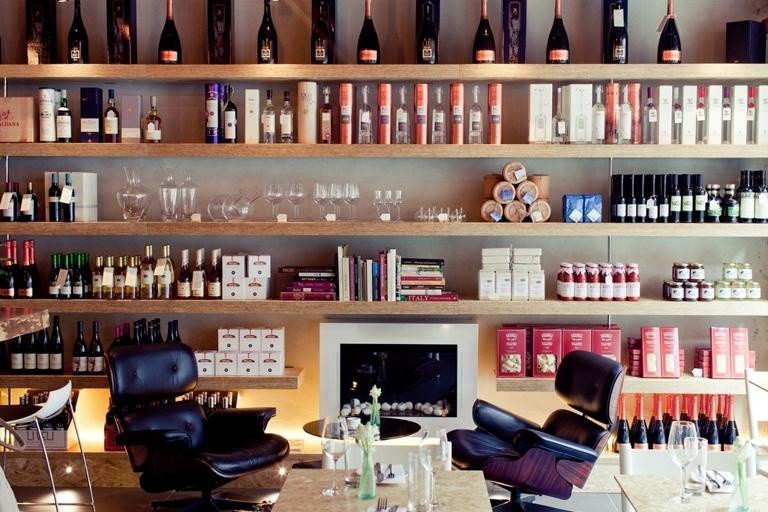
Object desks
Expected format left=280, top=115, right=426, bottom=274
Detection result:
left=265, top=464, right=493, bottom=511
left=609, top=468, right=767, bottom=511
left=299, top=415, right=423, bottom=442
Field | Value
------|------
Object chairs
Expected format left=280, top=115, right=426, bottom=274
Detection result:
left=444, top=349, right=628, bottom=511
left=98, top=336, right=291, bottom=511
left=0, top=378, right=96, bottom=511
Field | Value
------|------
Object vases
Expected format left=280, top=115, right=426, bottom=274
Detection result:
left=733, top=462, right=751, bottom=511
left=358, top=444, right=378, bottom=499
left=369, top=398, right=381, bottom=426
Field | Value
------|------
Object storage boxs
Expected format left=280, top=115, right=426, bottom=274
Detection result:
left=12, top=386, right=81, bottom=451
left=0, top=93, right=38, bottom=146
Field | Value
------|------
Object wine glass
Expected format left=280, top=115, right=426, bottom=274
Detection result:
left=422, top=427, right=451, bottom=508
left=321, top=416, right=345, bottom=498
left=260, top=180, right=405, bottom=222
left=666, top=419, right=699, bottom=506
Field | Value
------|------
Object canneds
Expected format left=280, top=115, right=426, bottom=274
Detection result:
left=663, top=262, right=761, bottom=301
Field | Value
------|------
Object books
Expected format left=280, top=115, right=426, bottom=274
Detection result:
left=278, top=244, right=459, bottom=301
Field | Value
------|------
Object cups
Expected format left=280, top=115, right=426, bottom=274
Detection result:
left=407, top=447, right=433, bottom=510
left=344, top=432, right=367, bottom=491
left=683, top=436, right=708, bottom=497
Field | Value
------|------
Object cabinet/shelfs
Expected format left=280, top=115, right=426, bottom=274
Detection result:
left=0, top=63, right=768, bottom=495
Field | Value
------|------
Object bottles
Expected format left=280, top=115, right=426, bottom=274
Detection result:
left=0, top=239, right=38, bottom=300
left=158, top=0, right=183, bottom=64
left=544, top=0, right=571, bottom=64
left=156, top=167, right=202, bottom=222
left=611, top=2, right=628, bottom=63
left=256, top=1, right=279, bottom=65
left=613, top=393, right=742, bottom=453
left=0, top=312, right=180, bottom=376
left=657, top=1, right=683, bottom=65
left=0, top=181, right=39, bottom=222
left=183, top=390, right=234, bottom=411
left=64, top=0, right=93, bottom=66
left=412, top=206, right=466, bottom=222
left=207, top=187, right=262, bottom=222
left=471, top=0, right=497, bottom=64
left=352, top=0, right=381, bottom=63
left=106, top=397, right=115, bottom=425
left=612, top=166, right=768, bottom=224
left=48, top=166, right=151, bottom=222
left=555, top=260, right=644, bottom=300
left=39, top=86, right=165, bottom=144
left=48, top=242, right=223, bottom=301
left=417, top=2, right=441, bottom=65
left=309, top=0, right=334, bottom=66
left=201, top=80, right=505, bottom=145
left=543, top=84, right=756, bottom=147
left=14, top=388, right=76, bottom=431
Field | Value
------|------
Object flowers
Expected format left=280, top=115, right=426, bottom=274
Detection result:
left=730, top=437, right=755, bottom=500
left=352, top=421, right=382, bottom=482
left=368, top=383, right=383, bottom=421
